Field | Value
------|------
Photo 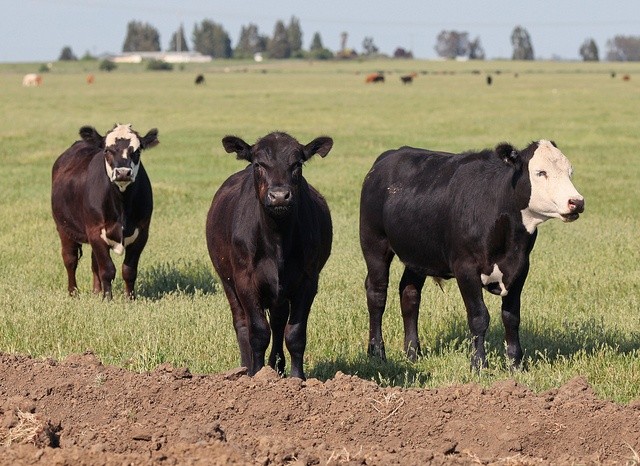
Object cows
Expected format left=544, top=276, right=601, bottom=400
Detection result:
left=194, top=74, right=204, bottom=85
left=360, top=139, right=584, bottom=370
left=401, top=75, right=413, bottom=83
left=51, top=121, right=160, bottom=300
left=205, top=130, right=334, bottom=381
left=366, top=72, right=378, bottom=82
left=23, top=74, right=42, bottom=88
